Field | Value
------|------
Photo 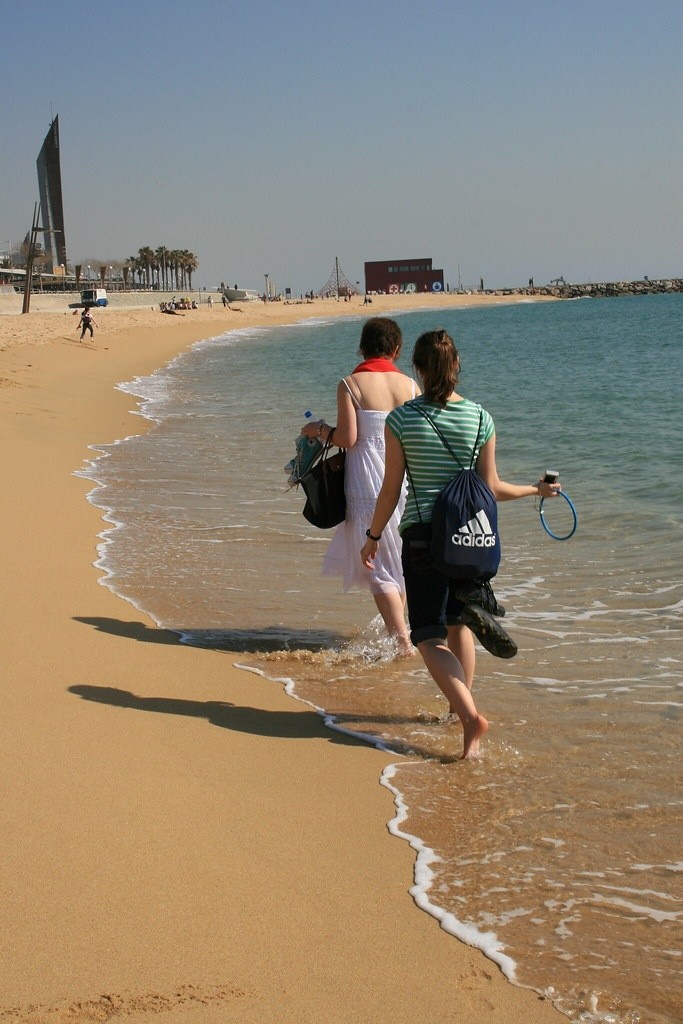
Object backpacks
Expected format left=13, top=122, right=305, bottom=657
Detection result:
left=396, top=402, right=500, bottom=583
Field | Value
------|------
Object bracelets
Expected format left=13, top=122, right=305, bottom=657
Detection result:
left=318, top=422, right=327, bottom=437
left=365, top=528, right=381, bottom=541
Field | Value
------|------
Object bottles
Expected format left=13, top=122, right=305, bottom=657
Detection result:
left=305, top=411, right=328, bottom=446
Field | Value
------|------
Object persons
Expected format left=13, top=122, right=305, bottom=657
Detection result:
left=360, top=330, right=562, bottom=759
left=76, top=306, right=98, bottom=343
left=227, top=284, right=238, bottom=290
left=262, top=283, right=427, bottom=306
left=284, top=316, right=422, bottom=660
left=159, top=296, right=227, bottom=314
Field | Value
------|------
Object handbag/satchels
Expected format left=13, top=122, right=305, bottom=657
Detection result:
left=299, top=428, right=348, bottom=528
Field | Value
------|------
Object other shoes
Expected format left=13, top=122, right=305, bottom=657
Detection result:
left=90, top=338, right=94, bottom=342
left=80, top=340, right=83, bottom=342
left=462, top=604, right=517, bottom=658
left=454, top=581, right=505, bottom=617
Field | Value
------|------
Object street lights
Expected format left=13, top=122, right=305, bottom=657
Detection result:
left=128, top=267, right=131, bottom=290
left=109, top=265, right=112, bottom=289
left=60, top=263, right=64, bottom=292
left=142, top=268, right=146, bottom=290
left=87, top=264, right=91, bottom=289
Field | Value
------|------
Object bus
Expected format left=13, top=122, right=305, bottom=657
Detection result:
left=79, top=288, right=108, bottom=307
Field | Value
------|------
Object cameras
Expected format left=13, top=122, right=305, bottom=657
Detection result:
left=543, top=470, right=559, bottom=484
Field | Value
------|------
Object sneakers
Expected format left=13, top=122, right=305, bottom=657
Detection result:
left=288, top=434, right=323, bottom=487
left=284, top=456, right=294, bottom=475
